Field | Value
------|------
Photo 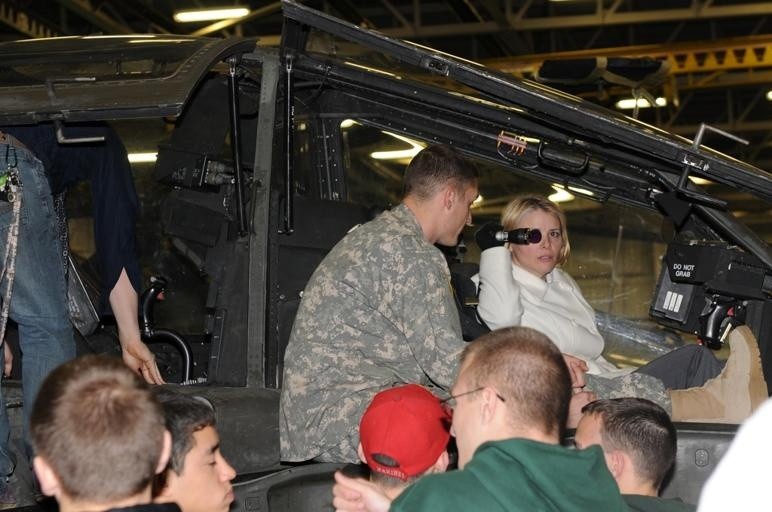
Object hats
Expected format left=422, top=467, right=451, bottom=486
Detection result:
left=358, top=383, right=454, bottom=481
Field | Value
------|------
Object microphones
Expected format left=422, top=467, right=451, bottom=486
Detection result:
left=487, top=229, right=540, bottom=244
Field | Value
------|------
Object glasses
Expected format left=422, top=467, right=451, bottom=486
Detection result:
left=438, top=385, right=506, bottom=419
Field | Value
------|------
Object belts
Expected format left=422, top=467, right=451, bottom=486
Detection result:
left=0, top=131, right=27, bottom=150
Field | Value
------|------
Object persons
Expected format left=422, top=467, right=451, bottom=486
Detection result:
left=2, top=114, right=169, bottom=512
left=333, top=326, right=628, bottom=512
left=571, top=398, right=693, bottom=511
left=473, top=194, right=721, bottom=389
left=359, top=385, right=452, bottom=501
left=275, top=146, right=769, bottom=467
left=29, top=352, right=179, bottom=512
left=144, top=386, right=236, bottom=512
